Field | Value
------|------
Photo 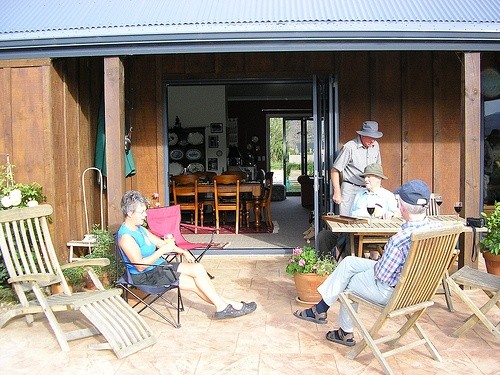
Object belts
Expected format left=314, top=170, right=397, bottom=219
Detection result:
left=341, top=180, right=366, bottom=188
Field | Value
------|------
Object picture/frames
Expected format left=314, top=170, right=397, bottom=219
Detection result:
left=208, top=158, right=218, bottom=170
left=209, top=135, right=218, bottom=148
left=210, top=123, right=223, bottom=133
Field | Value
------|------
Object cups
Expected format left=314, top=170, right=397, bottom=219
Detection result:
left=164, top=234, right=174, bottom=240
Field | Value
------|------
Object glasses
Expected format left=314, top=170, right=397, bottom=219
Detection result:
left=133, top=210, right=146, bottom=214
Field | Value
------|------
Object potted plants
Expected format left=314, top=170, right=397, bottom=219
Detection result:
left=477, top=200, right=500, bottom=276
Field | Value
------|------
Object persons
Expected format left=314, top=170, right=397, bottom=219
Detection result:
left=483, top=129, right=500, bottom=205
left=318, top=121, right=402, bottom=262
left=294, top=179, right=431, bottom=346
left=118, top=192, right=258, bottom=320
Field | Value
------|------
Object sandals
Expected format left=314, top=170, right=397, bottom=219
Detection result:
left=293, top=305, right=328, bottom=324
left=326, top=327, right=356, bottom=346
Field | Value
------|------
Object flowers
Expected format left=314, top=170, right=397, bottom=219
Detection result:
left=286, top=245, right=338, bottom=276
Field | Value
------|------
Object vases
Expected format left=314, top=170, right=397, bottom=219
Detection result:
left=294, top=272, right=330, bottom=302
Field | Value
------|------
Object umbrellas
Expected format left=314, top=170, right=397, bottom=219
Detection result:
left=95, top=90, right=137, bottom=190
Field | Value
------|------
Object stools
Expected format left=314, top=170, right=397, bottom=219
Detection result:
left=271, top=184, right=286, bottom=201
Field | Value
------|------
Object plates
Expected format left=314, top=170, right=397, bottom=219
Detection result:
left=167, top=131, right=204, bottom=172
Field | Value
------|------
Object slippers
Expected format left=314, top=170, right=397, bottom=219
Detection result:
left=214, top=301, right=257, bottom=319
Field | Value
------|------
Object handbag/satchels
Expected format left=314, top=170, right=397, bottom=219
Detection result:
left=465, top=217, right=486, bottom=228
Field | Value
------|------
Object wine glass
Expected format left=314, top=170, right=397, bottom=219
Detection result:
left=454, top=202, right=463, bottom=224
left=367, top=203, right=376, bottom=224
left=436, top=196, right=442, bottom=218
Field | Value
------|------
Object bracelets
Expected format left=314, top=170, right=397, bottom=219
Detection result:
left=181, top=250, right=185, bottom=255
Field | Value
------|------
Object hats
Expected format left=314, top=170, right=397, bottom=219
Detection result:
left=393, top=179, right=430, bottom=207
left=356, top=120, right=383, bottom=139
left=358, top=162, right=388, bottom=180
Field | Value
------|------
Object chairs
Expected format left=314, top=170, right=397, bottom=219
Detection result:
left=339, top=194, right=463, bottom=375
left=170, top=172, right=274, bottom=235
left=446, top=265, right=500, bottom=340
left=0, top=204, right=158, bottom=360
left=114, top=204, right=224, bottom=329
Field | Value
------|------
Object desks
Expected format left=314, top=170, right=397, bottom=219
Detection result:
left=170, top=184, right=263, bottom=232
left=323, top=215, right=491, bottom=294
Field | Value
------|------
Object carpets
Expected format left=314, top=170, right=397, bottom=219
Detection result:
left=180, top=221, right=280, bottom=235
